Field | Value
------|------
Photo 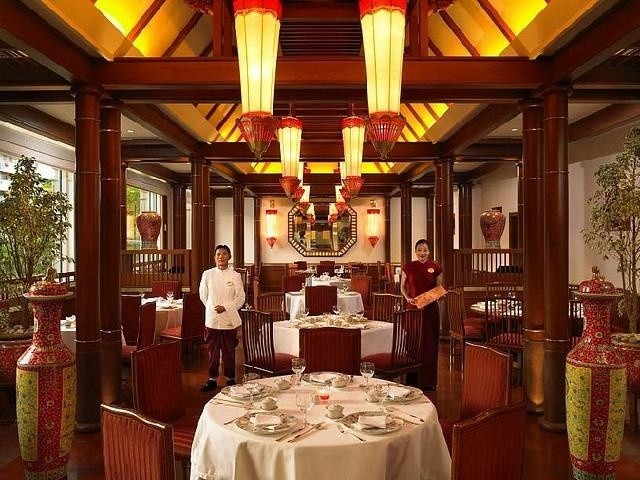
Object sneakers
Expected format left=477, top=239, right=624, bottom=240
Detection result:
left=200, top=380, right=216, bottom=392
left=225, top=379, right=235, bottom=386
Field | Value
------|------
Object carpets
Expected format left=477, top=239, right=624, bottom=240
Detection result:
left=0, top=431, right=106, bottom=479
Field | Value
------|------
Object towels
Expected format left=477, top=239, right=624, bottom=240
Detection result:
left=357, top=414, right=387, bottom=428
left=249, top=413, right=280, bottom=428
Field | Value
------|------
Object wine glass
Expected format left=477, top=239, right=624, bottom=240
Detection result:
left=167, top=291, right=173, bottom=302
left=291, top=358, right=306, bottom=387
left=355, top=311, right=362, bottom=321
left=302, top=307, right=308, bottom=317
left=343, top=283, right=348, bottom=294
left=243, top=373, right=260, bottom=409
left=296, top=393, right=313, bottom=430
left=333, top=305, right=340, bottom=315
left=360, top=362, right=375, bottom=393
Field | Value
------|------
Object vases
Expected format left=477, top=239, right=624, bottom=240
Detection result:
left=563, top=265, right=628, bottom=480
left=479, top=210, right=506, bottom=249
left=136, top=211, right=161, bottom=250
left=15, top=268, right=77, bottom=480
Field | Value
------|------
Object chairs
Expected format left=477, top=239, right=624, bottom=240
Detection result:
left=437, top=341, right=514, bottom=455
left=99, top=403, right=178, bottom=479
left=451, top=401, right=527, bottom=479
left=131, top=340, right=198, bottom=479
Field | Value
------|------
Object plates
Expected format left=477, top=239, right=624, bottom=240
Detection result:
left=365, top=397, right=379, bottom=403
left=343, top=412, right=404, bottom=434
left=325, top=414, right=345, bottom=419
left=221, top=384, right=274, bottom=402
left=311, top=371, right=344, bottom=383
left=161, top=305, right=176, bottom=309
left=236, top=413, right=296, bottom=435
left=259, top=405, right=278, bottom=410
left=364, top=383, right=423, bottom=401
left=349, top=291, right=360, bottom=295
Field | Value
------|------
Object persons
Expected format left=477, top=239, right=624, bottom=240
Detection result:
left=198, top=245, right=247, bottom=391
left=399, top=239, right=447, bottom=392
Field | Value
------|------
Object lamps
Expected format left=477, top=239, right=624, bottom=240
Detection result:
left=357, top=0, right=409, bottom=160
left=277, top=102, right=367, bottom=227
left=264, top=200, right=277, bottom=249
left=233, top=0, right=283, bottom=159
left=367, top=198, right=379, bottom=249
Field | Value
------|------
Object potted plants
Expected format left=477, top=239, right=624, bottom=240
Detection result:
left=0, top=153, right=75, bottom=388
left=579, top=125, right=640, bottom=388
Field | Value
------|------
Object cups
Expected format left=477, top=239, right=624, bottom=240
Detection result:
left=261, top=398, right=276, bottom=409
left=274, top=379, right=291, bottom=390
left=330, top=377, right=346, bottom=387
left=365, top=388, right=377, bottom=400
left=318, top=385, right=330, bottom=400
left=64, top=321, right=70, bottom=328
left=325, top=406, right=344, bottom=418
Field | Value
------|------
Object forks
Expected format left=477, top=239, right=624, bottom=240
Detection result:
left=336, top=424, right=366, bottom=442
